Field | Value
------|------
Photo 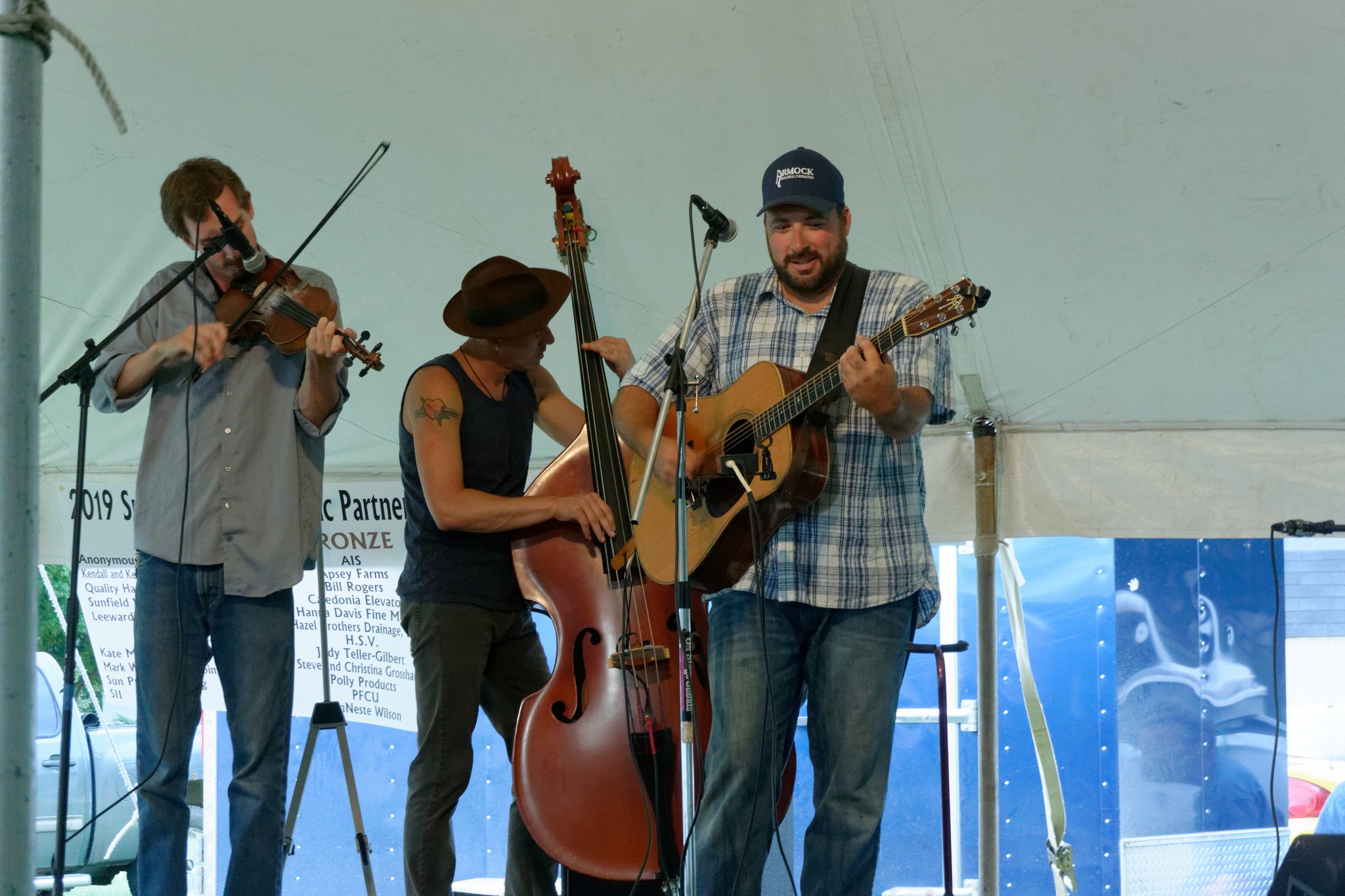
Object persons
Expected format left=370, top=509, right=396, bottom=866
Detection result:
left=613, top=144, right=960, bottom=896
left=398, top=256, right=637, bottom=896
left=91, top=156, right=358, bottom=896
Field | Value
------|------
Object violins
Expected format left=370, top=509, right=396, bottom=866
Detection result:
left=214, top=257, right=385, bottom=378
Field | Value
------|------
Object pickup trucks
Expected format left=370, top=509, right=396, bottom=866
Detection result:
left=36, top=652, right=204, bottom=896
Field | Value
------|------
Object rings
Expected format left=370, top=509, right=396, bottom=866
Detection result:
left=613, top=350, right=617, bottom=355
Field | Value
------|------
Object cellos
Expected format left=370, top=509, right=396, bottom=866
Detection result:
left=509, top=153, right=799, bottom=896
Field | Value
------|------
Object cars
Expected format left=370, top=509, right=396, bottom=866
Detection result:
left=1287, top=755, right=1345, bottom=847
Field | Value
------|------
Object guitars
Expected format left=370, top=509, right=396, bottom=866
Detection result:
left=625, top=273, right=992, bottom=596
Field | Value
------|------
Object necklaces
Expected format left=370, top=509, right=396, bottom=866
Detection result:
left=459, top=344, right=506, bottom=400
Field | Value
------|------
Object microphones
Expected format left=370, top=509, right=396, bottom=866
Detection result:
left=1272, top=519, right=1317, bottom=536
left=691, top=194, right=739, bottom=243
left=204, top=198, right=266, bottom=273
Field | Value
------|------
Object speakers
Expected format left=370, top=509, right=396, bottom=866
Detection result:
left=1266, top=833, right=1345, bottom=896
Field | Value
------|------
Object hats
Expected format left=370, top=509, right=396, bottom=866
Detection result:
left=443, top=255, right=573, bottom=338
left=756, top=146, right=845, bottom=217
